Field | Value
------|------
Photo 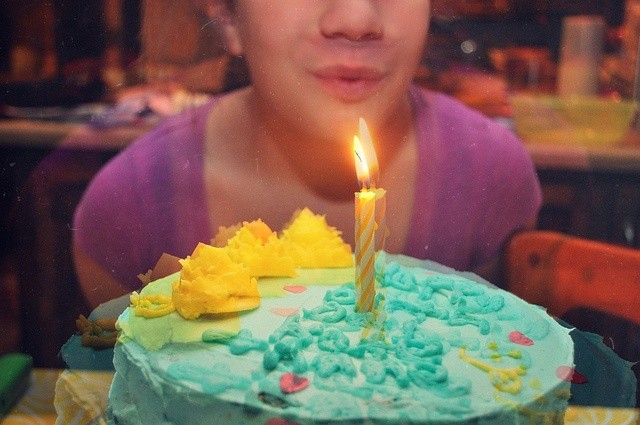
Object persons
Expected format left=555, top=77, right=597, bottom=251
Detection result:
left=72, top=0, right=542, bottom=300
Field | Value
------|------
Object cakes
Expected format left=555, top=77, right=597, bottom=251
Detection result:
left=105, top=252, right=574, bottom=425
